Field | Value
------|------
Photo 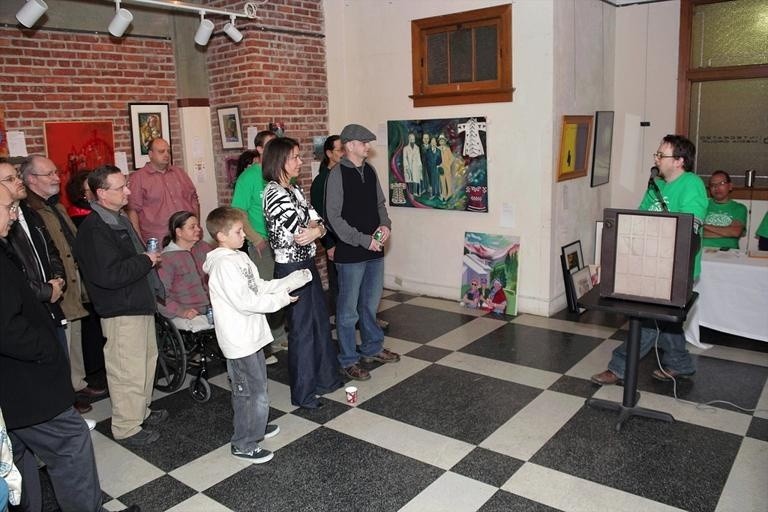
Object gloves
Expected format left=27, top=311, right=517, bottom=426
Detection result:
left=4, top=462, right=22, bottom=506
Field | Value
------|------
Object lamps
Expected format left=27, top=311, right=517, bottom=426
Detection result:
left=15, top=0, right=264, bottom=46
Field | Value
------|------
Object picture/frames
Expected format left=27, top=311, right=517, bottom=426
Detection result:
left=216, top=105, right=245, bottom=150
left=43, top=120, right=115, bottom=218
left=560, top=221, right=603, bottom=316
left=590, top=110, right=614, bottom=187
left=127, top=102, right=174, bottom=171
left=556, top=113, right=594, bottom=182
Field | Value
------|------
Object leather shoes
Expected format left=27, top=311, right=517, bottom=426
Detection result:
left=315, top=380, right=345, bottom=395
left=292, top=397, right=325, bottom=409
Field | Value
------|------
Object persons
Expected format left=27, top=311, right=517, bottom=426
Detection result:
left=703, top=171, right=747, bottom=249
left=590, top=134, right=709, bottom=385
left=202, top=206, right=313, bottom=464
left=262, top=139, right=345, bottom=408
left=230, top=131, right=295, bottom=280
left=754, top=210, right=768, bottom=251
left=402, top=132, right=454, bottom=201
left=311, top=135, right=389, bottom=330
left=65, top=134, right=217, bottom=448
left=0, top=154, right=103, bottom=512
left=322, top=124, right=400, bottom=380
left=463, top=278, right=507, bottom=314
left=236, top=149, right=260, bottom=178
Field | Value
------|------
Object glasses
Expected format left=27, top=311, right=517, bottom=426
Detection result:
left=1, top=204, right=15, bottom=212
left=32, top=170, right=57, bottom=177
left=709, top=181, right=727, bottom=187
left=653, top=153, right=673, bottom=161
left=107, top=182, right=130, bottom=191
left=1, top=174, right=20, bottom=182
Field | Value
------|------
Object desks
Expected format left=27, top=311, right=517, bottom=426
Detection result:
left=683, top=248, right=768, bottom=354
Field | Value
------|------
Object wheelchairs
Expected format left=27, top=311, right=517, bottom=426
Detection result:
left=148, top=305, right=232, bottom=405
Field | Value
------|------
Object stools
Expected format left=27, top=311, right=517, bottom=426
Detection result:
left=576, top=283, right=700, bottom=432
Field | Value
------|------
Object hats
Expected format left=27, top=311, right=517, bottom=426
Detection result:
left=437, top=135, right=448, bottom=143
left=341, top=124, right=376, bottom=144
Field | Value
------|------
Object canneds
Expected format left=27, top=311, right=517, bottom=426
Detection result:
left=376, top=231, right=383, bottom=249
left=146, top=238, right=159, bottom=253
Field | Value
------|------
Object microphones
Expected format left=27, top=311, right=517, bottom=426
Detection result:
left=648, top=166, right=659, bottom=185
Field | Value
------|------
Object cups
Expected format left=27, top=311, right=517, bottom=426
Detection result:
left=344, top=386, right=358, bottom=404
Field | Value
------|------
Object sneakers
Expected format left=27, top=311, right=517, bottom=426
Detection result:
left=231, top=443, right=274, bottom=464
left=256, top=425, right=280, bottom=443
left=74, top=385, right=108, bottom=432
left=265, top=340, right=289, bottom=365
left=115, top=430, right=160, bottom=446
left=360, top=349, right=400, bottom=364
left=143, top=409, right=169, bottom=425
left=376, top=318, right=390, bottom=329
left=338, top=364, right=371, bottom=381
left=651, top=367, right=695, bottom=381
left=591, top=370, right=625, bottom=386
